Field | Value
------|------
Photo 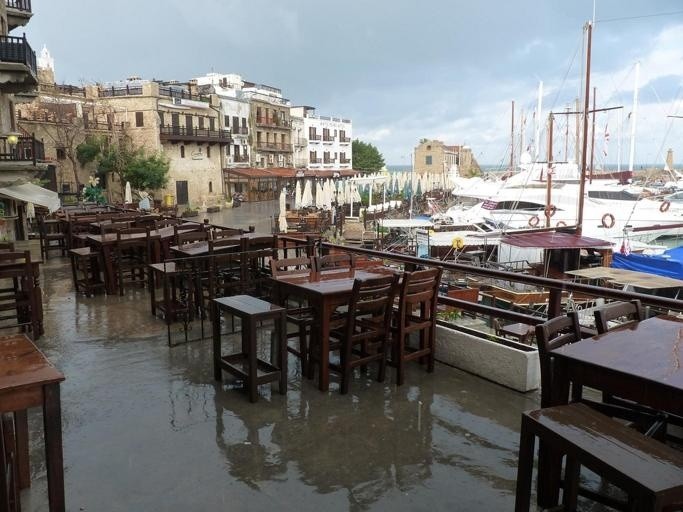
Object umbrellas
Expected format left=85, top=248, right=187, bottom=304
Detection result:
left=322, top=172, right=448, bottom=212
left=278, top=191, right=287, bottom=234
left=315, top=182, right=322, bottom=209
left=301, top=180, right=311, bottom=206
left=294, top=182, right=300, bottom=209
left=124, top=180, right=132, bottom=206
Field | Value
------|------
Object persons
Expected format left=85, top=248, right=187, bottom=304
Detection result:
left=138, top=191, right=149, bottom=210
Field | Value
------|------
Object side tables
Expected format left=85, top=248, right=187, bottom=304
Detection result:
left=212, top=294, right=288, bottom=403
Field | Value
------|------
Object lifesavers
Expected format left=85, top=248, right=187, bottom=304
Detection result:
left=660, top=201, right=670, bottom=212
left=602, top=213, right=615, bottom=228
left=545, top=206, right=555, bottom=217
left=528, top=216, right=539, bottom=226
left=556, top=221, right=568, bottom=227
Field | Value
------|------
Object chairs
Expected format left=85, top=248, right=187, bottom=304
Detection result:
left=149, top=214, right=278, bottom=323
left=65, top=207, right=149, bottom=298
left=0, top=240, right=45, bottom=340
left=593, top=299, right=681, bottom=422
left=38, top=207, right=65, bottom=260
left=269, top=253, right=442, bottom=394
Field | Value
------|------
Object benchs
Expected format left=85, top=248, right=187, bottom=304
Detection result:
left=515, top=400, right=682, bottom=510
